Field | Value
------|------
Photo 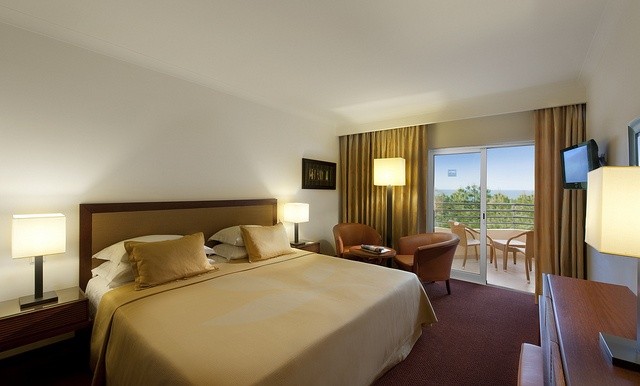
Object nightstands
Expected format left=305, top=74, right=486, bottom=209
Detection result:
left=0, top=286, right=90, bottom=384
left=293, top=241, right=320, bottom=255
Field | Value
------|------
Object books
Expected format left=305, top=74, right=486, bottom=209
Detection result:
left=360, top=244, right=391, bottom=255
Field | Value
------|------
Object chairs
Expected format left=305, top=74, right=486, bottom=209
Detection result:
left=449, top=219, right=498, bottom=269
left=504, top=231, right=534, bottom=283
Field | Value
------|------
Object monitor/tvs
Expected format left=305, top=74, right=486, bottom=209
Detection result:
left=560, top=139, right=599, bottom=189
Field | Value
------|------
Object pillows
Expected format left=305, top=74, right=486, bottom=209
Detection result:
left=91, top=234, right=216, bottom=268
left=240, top=222, right=295, bottom=263
left=210, top=244, right=249, bottom=262
left=207, top=224, right=262, bottom=247
left=124, top=231, right=220, bottom=291
left=91, top=257, right=215, bottom=287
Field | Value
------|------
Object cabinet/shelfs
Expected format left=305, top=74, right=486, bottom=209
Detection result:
left=517, top=271, right=640, bottom=386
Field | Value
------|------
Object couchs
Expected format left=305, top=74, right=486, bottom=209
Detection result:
left=394, top=231, right=459, bottom=295
left=333, top=223, right=382, bottom=264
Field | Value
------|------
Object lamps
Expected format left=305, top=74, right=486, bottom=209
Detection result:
left=373, top=158, right=406, bottom=267
left=284, top=203, right=310, bottom=246
left=582, top=166, right=640, bottom=373
left=11, top=212, right=66, bottom=309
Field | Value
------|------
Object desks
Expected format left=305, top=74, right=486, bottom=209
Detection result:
left=490, top=238, right=526, bottom=269
left=348, top=245, right=396, bottom=268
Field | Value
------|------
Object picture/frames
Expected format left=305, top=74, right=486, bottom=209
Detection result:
left=302, top=158, right=337, bottom=190
left=627, top=118, right=640, bottom=166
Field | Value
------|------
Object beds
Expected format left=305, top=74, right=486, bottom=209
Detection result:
left=79, top=197, right=438, bottom=386
